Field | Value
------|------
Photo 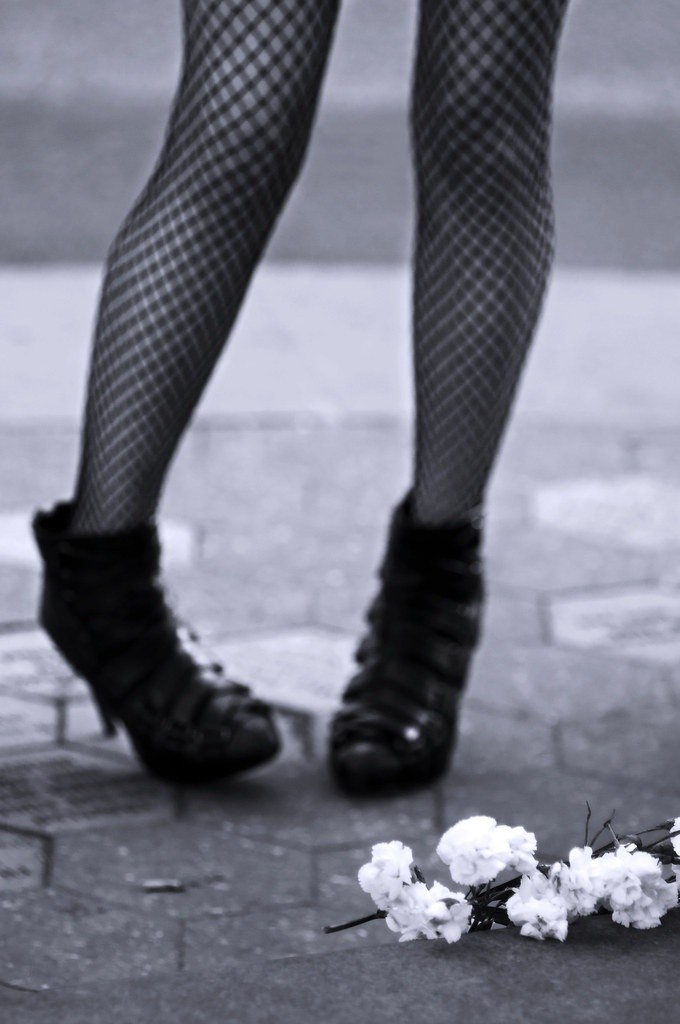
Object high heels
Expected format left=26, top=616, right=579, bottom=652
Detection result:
left=32, top=500, right=282, bottom=788
left=330, top=489, right=486, bottom=800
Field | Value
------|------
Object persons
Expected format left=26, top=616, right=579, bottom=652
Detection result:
left=30, top=0, right=569, bottom=804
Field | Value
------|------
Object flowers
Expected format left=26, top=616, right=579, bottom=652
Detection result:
left=320, top=799, right=680, bottom=946
